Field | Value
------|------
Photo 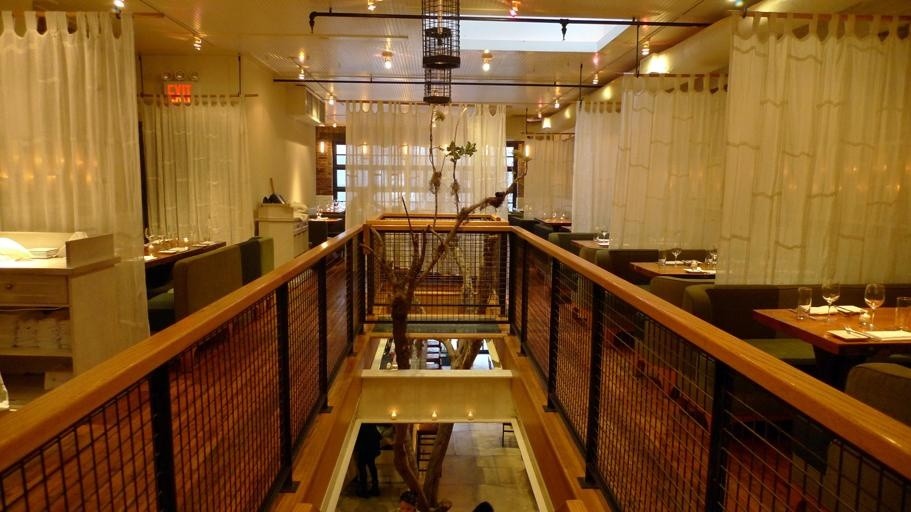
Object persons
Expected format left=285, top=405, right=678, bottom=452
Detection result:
left=394, top=490, right=420, bottom=511
left=352, top=423, right=383, bottom=497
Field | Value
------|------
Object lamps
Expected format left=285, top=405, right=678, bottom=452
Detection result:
left=420, top=0, right=461, bottom=108
left=159, top=69, right=201, bottom=105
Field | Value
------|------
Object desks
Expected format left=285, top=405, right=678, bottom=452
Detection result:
left=754, top=304, right=906, bottom=368
left=567, top=236, right=613, bottom=251
left=536, top=214, right=575, bottom=231
left=632, top=259, right=722, bottom=280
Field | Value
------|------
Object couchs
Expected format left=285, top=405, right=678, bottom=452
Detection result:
left=550, top=234, right=584, bottom=306
left=836, top=360, right=907, bottom=512
left=147, top=235, right=275, bottom=374
left=688, top=282, right=819, bottom=412
left=638, top=277, right=683, bottom=391
left=595, top=245, right=671, bottom=347
left=576, top=249, right=595, bottom=315
left=509, top=213, right=535, bottom=282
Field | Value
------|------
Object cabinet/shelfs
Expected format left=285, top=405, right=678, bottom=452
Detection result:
left=254, top=202, right=311, bottom=278
left=0, top=230, right=124, bottom=418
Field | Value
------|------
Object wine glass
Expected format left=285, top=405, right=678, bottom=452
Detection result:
left=865, top=283, right=885, bottom=330
left=672, top=240, right=681, bottom=268
left=820, top=279, right=840, bottom=321
left=144, top=228, right=156, bottom=249
left=601, top=225, right=608, bottom=240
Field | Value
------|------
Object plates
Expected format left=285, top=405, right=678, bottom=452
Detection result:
left=836, top=304, right=865, bottom=315
left=685, top=268, right=716, bottom=273
left=665, top=259, right=701, bottom=265
left=825, top=330, right=869, bottom=341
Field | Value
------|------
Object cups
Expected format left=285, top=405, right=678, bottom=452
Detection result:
left=894, top=296, right=911, bottom=331
left=658, top=256, right=667, bottom=267
left=796, top=286, right=812, bottom=320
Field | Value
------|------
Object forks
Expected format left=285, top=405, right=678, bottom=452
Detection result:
left=843, top=325, right=877, bottom=340
